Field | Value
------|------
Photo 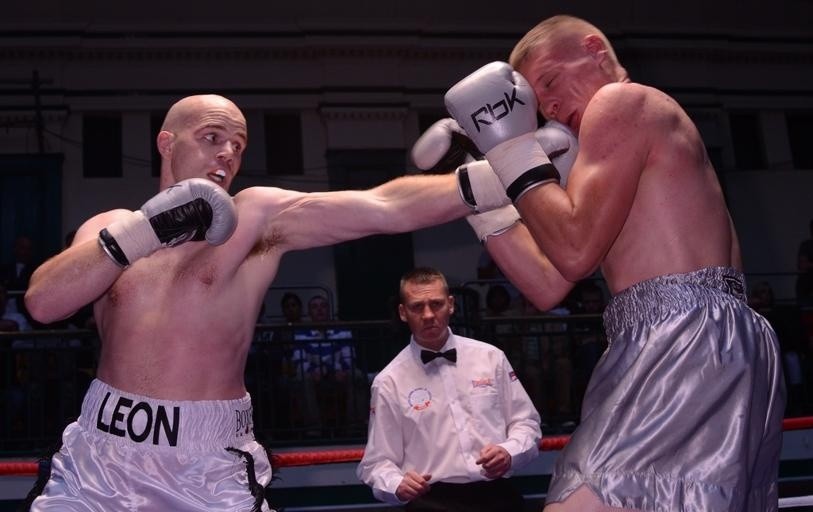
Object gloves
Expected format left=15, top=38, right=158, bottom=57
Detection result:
left=444, top=61, right=560, bottom=206
left=454, top=120, right=578, bottom=215
left=97, top=178, right=239, bottom=271
left=411, top=118, right=522, bottom=243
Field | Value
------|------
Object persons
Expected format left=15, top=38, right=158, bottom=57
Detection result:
left=252, top=293, right=358, bottom=440
left=357, top=264, right=541, bottom=512
left=414, top=13, right=783, bottom=512
left=443, top=282, right=802, bottom=425
left=794, top=224, right=813, bottom=415
left=22, top=92, right=578, bottom=512
left=1, top=226, right=102, bottom=452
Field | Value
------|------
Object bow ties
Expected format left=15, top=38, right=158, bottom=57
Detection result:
left=421, top=348, right=457, bottom=365
